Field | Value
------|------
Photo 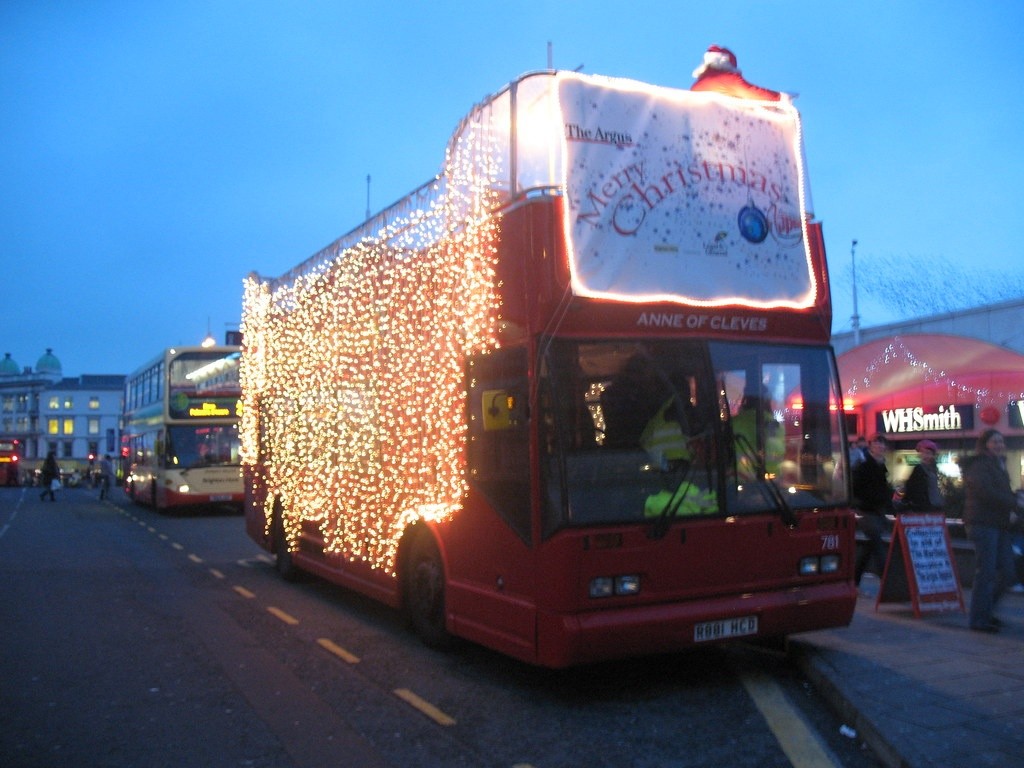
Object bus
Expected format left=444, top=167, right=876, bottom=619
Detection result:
left=122, top=344, right=246, bottom=517
left=242, top=35, right=859, bottom=674
left=0, top=438, right=23, bottom=488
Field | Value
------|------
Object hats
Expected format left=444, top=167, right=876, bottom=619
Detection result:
left=916, top=440, right=937, bottom=453
left=868, top=433, right=886, bottom=445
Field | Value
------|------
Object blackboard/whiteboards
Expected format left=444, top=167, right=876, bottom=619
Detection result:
left=874, top=514, right=963, bottom=601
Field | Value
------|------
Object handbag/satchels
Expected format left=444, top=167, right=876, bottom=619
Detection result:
left=50, top=479, right=60, bottom=490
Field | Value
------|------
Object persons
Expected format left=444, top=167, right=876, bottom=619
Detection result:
left=903, top=439, right=947, bottom=518
left=640, top=364, right=720, bottom=473
left=848, top=435, right=912, bottom=599
left=39, top=450, right=62, bottom=502
left=957, top=430, right=1024, bottom=633
left=99, top=453, right=116, bottom=500
left=731, top=384, right=786, bottom=482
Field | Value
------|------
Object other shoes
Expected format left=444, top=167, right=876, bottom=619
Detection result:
left=855, top=587, right=867, bottom=597
left=990, top=615, right=1005, bottom=626
left=971, top=624, right=998, bottom=633
left=50, top=498, right=54, bottom=501
left=39, top=494, right=43, bottom=501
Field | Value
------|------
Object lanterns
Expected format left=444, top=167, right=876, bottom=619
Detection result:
left=978, top=405, right=1001, bottom=424
